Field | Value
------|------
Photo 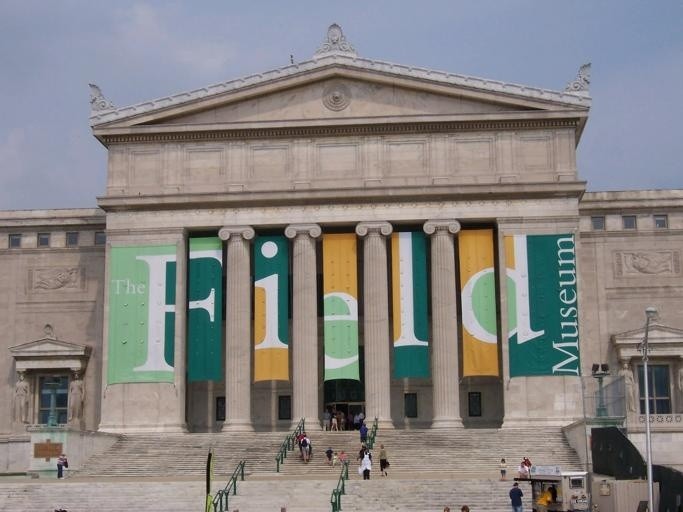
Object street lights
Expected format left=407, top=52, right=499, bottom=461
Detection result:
left=643, top=306, right=658, bottom=512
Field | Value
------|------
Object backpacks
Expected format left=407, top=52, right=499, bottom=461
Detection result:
left=301, top=438, right=307, bottom=447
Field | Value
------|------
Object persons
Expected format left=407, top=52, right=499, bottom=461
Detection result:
left=321, top=407, right=365, bottom=432
left=518, top=462, right=529, bottom=478
left=509, top=482, right=523, bottom=512
left=360, top=423, right=368, bottom=447
left=295, top=431, right=313, bottom=463
left=500, top=458, right=507, bottom=480
left=15, top=374, right=30, bottom=422
left=536, top=486, right=554, bottom=512
left=68, top=374, right=85, bottom=421
left=551, top=484, right=557, bottom=502
left=523, top=456, right=533, bottom=479
left=443, top=507, right=450, bottom=512
left=56, top=452, right=68, bottom=479
left=461, top=505, right=469, bottom=512
left=324, top=444, right=388, bottom=480
